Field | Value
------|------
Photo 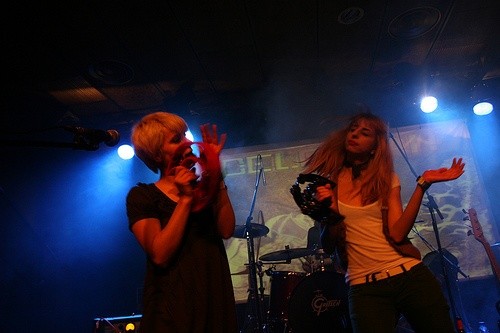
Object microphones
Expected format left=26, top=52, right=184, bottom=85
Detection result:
left=261, top=211, right=268, bottom=238
left=260, top=155, right=266, bottom=186
left=67, top=126, right=120, bottom=146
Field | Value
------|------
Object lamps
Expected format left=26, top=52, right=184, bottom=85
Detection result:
left=60, top=114, right=122, bottom=152
left=469, top=80, right=494, bottom=116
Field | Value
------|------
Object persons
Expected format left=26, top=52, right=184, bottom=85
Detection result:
left=292, top=113, right=465, bottom=333
left=302, top=220, right=336, bottom=274
left=125, top=111, right=240, bottom=333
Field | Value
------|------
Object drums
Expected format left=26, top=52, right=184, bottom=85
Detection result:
left=264, top=268, right=351, bottom=332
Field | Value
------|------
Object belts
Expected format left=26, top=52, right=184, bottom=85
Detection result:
left=348, top=258, right=423, bottom=287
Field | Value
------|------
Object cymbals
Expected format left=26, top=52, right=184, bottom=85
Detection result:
left=230, top=223, right=269, bottom=240
left=256, top=248, right=309, bottom=263
left=289, top=173, right=346, bottom=226
left=230, top=269, right=260, bottom=275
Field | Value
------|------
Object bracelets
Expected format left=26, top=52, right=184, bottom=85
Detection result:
left=416, top=175, right=429, bottom=192
left=214, top=173, right=228, bottom=192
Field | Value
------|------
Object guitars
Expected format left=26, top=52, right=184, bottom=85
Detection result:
left=461, top=205, right=500, bottom=284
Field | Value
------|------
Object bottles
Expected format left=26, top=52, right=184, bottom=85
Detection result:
left=477, top=322, right=489, bottom=333
